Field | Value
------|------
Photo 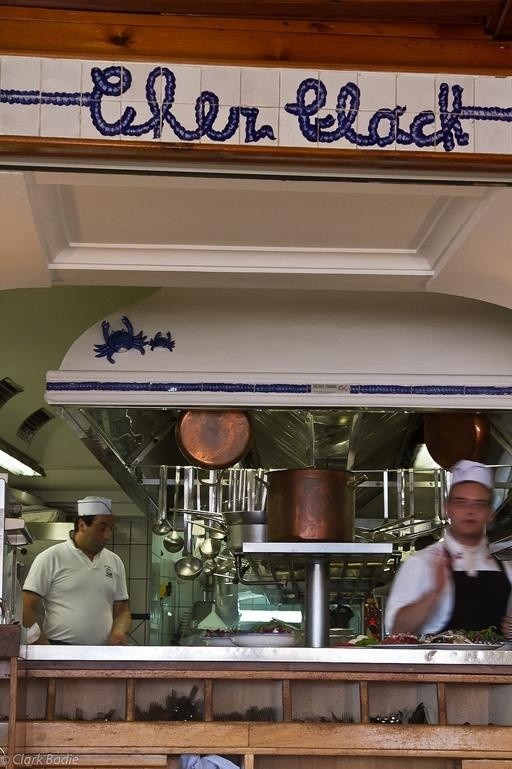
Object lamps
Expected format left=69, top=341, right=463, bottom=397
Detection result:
left=0, top=437, right=46, bottom=477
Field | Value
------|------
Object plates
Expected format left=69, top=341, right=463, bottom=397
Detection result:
left=198, top=631, right=303, bottom=647
left=364, top=639, right=504, bottom=649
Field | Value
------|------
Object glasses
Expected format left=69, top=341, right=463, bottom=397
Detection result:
left=452, top=500, right=489, bottom=510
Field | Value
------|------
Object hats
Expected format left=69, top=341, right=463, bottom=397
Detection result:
left=451, top=460, right=495, bottom=493
left=77, top=496, right=112, bottom=517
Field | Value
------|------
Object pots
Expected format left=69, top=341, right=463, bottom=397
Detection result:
left=420, top=414, right=493, bottom=473
left=174, top=409, right=255, bottom=488
left=251, top=467, right=370, bottom=542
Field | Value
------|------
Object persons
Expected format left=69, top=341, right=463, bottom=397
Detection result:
left=22, top=494, right=133, bottom=646
left=381, top=459, right=511, bottom=643
